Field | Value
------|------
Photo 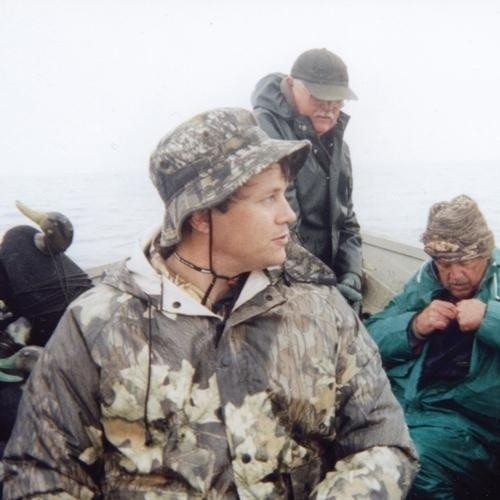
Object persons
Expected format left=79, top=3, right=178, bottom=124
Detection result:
left=360, top=196, right=500, bottom=500
left=250, top=47, right=364, bottom=319
left=0, top=107, right=420, bottom=500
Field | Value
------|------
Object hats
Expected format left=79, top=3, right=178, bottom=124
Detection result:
left=149, top=108, right=312, bottom=247
left=421, top=195, right=495, bottom=263
left=292, top=48, right=359, bottom=101
